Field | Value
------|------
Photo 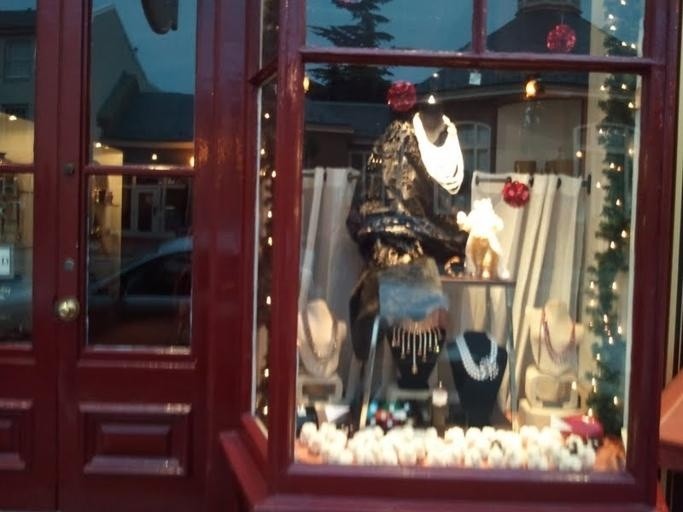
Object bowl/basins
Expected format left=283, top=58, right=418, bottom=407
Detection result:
left=413, top=112, right=464, bottom=195
left=456, top=333, right=499, bottom=382
left=302, top=309, right=339, bottom=365
left=390, top=308, right=444, bottom=375
